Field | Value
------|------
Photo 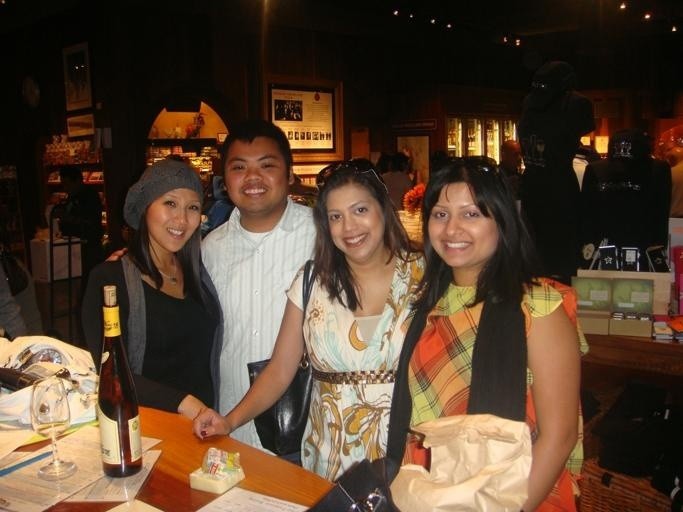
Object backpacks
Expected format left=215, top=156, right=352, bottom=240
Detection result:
left=246, top=258, right=322, bottom=452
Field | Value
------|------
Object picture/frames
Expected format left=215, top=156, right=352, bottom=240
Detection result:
left=262, top=72, right=345, bottom=163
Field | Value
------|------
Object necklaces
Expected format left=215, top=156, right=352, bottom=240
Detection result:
left=153, top=254, right=178, bottom=286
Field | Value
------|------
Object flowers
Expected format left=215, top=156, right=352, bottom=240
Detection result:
left=402, top=181, right=426, bottom=213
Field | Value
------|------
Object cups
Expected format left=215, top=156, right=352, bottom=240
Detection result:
left=30, top=377, right=77, bottom=483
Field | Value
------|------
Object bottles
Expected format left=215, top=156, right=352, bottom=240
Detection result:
left=100, top=287, right=144, bottom=478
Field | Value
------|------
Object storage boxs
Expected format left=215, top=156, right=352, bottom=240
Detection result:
left=577, top=266, right=672, bottom=316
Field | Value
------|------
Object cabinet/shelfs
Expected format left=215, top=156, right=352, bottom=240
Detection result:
left=43, top=144, right=110, bottom=228
left=143, top=137, right=223, bottom=201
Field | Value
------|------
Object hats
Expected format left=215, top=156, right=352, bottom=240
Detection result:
left=124, top=158, right=203, bottom=228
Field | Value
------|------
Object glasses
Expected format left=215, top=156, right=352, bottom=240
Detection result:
left=318, top=158, right=388, bottom=188
left=430, top=157, right=500, bottom=177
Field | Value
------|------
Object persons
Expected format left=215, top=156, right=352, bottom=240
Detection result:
left=0, top=253, right=44, bottom=339
left=193, top=156, right=428, bottom=482
left=665, top=148, right=682, bottom=217
left=368, top=150, right=415, bottom=210
left=80, top=157, right=224, bottom=419
left=581, top=130, right=669, bottom=248
left=497, top=141, right=524, bottom=200
left=198, top=119, right=318, bottom=465
left=383, top=157, right=589, bottom=512
left=58, top=165, right=108, bottom=275
